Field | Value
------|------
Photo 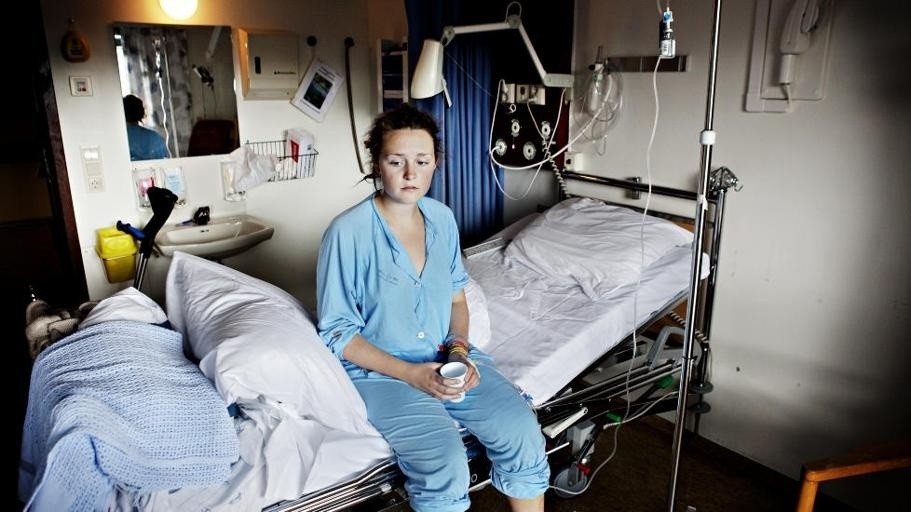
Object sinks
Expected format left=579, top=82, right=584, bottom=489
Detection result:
left=155, top=218, right=274, bottom=260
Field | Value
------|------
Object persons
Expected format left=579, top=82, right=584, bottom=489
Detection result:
left=123, top=93, right=170, bottom=160
left=318, top=102, right=550, bottom=511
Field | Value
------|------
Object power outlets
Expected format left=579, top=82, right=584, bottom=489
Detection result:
left=529, top=84, right=545, bottom=106
left=500, top=82, right=514, bottom=104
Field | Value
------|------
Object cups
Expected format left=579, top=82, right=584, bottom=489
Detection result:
left=440, top=362, right=469, bottom=404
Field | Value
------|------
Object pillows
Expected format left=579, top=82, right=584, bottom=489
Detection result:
left=506, top=195, right=697, bottom=302
left=166, top=250, right=372, bottom=433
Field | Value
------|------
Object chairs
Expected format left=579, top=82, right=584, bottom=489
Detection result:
left=798, top=436, right=911, bottom=511
left=187, top=117, right=235, bottom=157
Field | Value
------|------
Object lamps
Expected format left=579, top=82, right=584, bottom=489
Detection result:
left=409, top=16, right=577, bottom=101
left=158, top=0, right=198, bottom=19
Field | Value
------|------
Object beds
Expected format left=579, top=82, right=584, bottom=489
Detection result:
left=20, top=166, right=743, bottom=511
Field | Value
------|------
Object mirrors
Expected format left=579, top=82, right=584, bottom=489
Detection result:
left=110, top=20, right=238, bottom=162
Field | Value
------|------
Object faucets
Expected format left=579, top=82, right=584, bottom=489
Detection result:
left=194, top=205, right=211, bottom=226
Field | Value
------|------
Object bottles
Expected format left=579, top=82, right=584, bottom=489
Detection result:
left=659, top=30, right=679, bottom=58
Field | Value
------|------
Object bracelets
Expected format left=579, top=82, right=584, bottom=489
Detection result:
left=445, top=332, right=470, bottom=357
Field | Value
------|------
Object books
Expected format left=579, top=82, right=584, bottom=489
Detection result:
left=286, top=127, right=316, bottom=178
